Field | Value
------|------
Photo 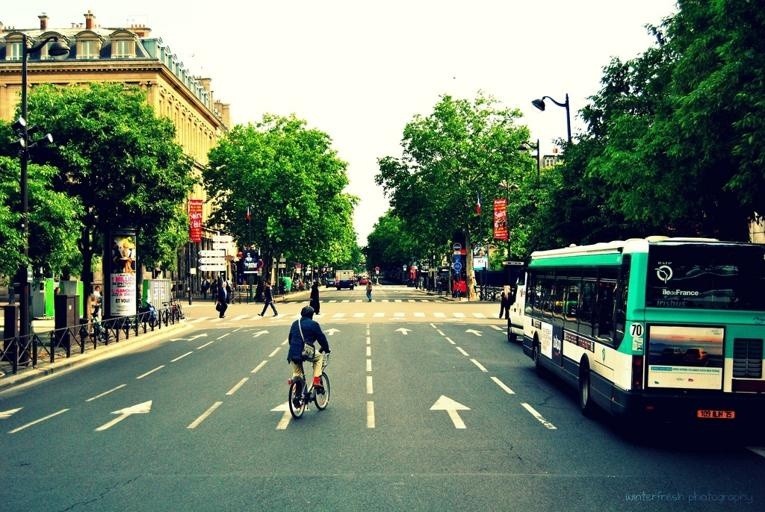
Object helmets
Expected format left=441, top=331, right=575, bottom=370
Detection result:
left=301, top=305, right=315, bottom=318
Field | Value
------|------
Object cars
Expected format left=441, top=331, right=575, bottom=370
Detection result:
left=326, top=269, right=369, bottom=290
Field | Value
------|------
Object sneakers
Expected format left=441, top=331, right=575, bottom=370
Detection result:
left=220, top=315, right=225, bottom=318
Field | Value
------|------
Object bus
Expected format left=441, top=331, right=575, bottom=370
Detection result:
left=523, top=234, right=765, bottom=430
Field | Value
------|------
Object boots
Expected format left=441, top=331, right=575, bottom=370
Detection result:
left=313, top=377, right=325, bottom=389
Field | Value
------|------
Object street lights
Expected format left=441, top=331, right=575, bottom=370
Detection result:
left=0, top=30, right=72, bottom=366
left=518, top=93, right=572, bottom=186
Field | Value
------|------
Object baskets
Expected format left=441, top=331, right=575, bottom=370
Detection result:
left=322, top=353, right=329, bottom=367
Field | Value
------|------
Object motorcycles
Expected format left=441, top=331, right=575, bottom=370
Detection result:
left=137, top=301, right=159, bottom=327
left=79, top=318, right=112, bottom=343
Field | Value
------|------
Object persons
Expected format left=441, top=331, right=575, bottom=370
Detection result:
left=366, top=278, right=373, bottom=302
left=499, top=285, right=512, bottom=320
left=287, top=306, right=330, bottom=409
left=54, top=287, right=60, bottom=295
left=93, top=285, right=101, bottom=318
left=201, top=278, right=320, bottom=318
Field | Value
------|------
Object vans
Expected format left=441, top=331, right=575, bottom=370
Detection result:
left=508, top=283, right=527, bottom=343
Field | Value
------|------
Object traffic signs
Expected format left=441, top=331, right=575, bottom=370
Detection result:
left=198, top=235, right=238, bottom=272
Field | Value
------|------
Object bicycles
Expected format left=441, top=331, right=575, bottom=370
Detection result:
left=162, top=298, right=184, bottom=325
left=288, top=347, right=331, bottom=420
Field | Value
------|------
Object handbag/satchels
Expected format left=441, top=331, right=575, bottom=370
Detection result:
left=216, top=303, right=223, bottom=312
left=300, top=343, right=316, bottom=360
